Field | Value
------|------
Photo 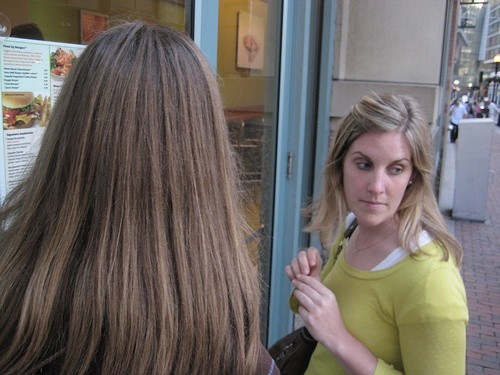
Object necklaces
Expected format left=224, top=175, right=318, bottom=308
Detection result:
left=354, top=222, right=400, bottom=254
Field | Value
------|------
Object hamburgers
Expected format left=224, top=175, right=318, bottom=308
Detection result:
left=1, top=92, right=37, bottom=130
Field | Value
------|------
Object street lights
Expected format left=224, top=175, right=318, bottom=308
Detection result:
left=454, top=79, right=458, bottom=85
left=490, top=54, right=500, bottom=102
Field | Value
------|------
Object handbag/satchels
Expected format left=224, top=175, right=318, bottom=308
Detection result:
left=266, top=326, right=317, bottom=375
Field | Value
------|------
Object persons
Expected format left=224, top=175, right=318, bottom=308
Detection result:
left=285, top=90, right=470, bottom=374
left=445, top=94, right=500, bottom=143
left=0, top=20, right=280, bottom=375
